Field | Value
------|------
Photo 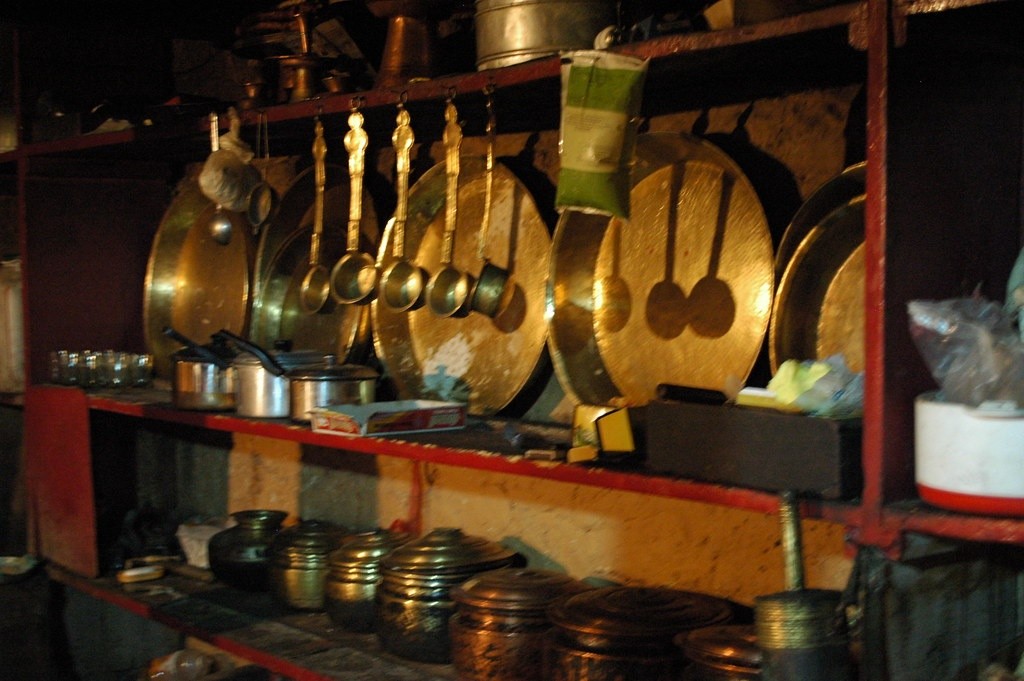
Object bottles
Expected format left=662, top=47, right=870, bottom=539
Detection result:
left=135, top=648, right=216, bottom=681
left=208, top=509, right=290, bottom=591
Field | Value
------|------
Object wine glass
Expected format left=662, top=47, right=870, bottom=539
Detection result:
left=46, top=348, right=154, bottom=402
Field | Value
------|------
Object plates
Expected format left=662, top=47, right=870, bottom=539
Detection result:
left=145, top=101, right=227, bottom=121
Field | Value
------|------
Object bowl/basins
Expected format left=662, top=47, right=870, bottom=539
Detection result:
left=251, top=161, right=398, bottom=370
left=548, top=131, right=777, bottom=407
left=767, top=157, right=868, bottom=377
left=370, top=153, right=550, bottom=421
left=143, top=167, right=259, bottom=375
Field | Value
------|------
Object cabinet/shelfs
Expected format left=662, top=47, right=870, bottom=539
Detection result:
left=0, top=0, right=1024, bottom=681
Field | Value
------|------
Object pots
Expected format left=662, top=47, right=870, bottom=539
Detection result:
left=233, top=340, right=325, bottom=418
left=446, top=568, right=578, bottom=681
left=218, top=330, right=381, bottom=422
left=543, top=586, right=766, bottom=681
left=325, top=529, right=414, bottom=630
left=381, top=528, right=530, bottom=666
left=160, top=326, right=245, bottom=410
left=270, top=519, right=352, bottom=609
left=473, top=1, right=608, bottom=72
